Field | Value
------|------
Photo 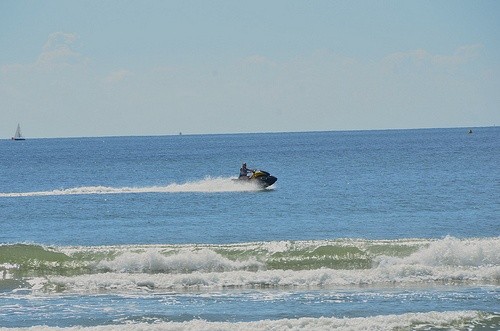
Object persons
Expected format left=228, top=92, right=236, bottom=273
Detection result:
left=239, top=163, right=254, bottom=180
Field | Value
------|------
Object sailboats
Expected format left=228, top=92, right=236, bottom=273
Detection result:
left=13, top=122, right=26, bottom=140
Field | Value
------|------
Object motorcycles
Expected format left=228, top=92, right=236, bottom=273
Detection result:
left=231, top=169, right=277, bottom=189
